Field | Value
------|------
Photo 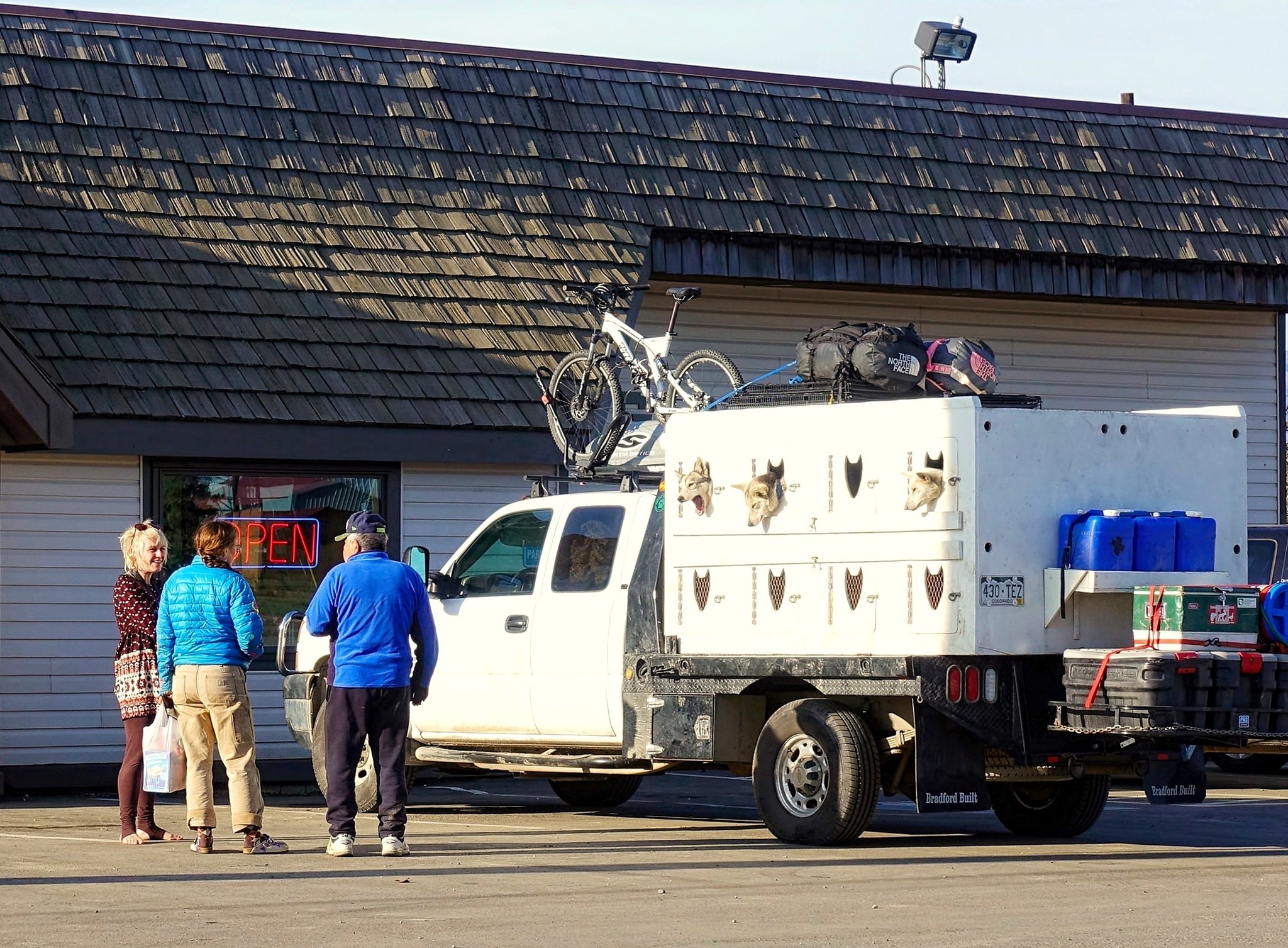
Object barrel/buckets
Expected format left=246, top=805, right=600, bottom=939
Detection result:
left=1055, top=507, right=1216, bottom=570
left=1245, top=583, right=1288, bottom=644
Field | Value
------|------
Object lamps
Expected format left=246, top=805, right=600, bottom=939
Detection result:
left=914, top=16, right=977, bottom=88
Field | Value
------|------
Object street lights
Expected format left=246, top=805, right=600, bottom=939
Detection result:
left=914, top=16, right=977, bottom=89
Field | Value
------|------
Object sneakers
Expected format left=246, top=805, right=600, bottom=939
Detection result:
left=243, top=832, right=288, bottom=854
left=381, top=833, right=410, bottom=857
left=190, top=836, right=214, bottom=854
left=326, top=834, right=354, bottom=856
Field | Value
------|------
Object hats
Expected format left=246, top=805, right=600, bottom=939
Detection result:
left=335, top=510, right=387, bottom=542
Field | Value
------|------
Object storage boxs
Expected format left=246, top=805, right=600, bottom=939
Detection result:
left=1062, top=646, right=1288, bottom=741
left=1133, top=585, right=1261, bottom=651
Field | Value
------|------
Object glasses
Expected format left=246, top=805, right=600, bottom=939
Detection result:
left=131, top=522, right=160, bottom=545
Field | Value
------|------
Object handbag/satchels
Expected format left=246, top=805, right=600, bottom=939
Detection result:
left=142, top=700, right=186, bottom=793
left=795, top=321, right=928, bottom=393
left=918, top=337, right=998, bottom=395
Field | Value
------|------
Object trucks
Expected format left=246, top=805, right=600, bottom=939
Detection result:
left=276, top=391, right=1288, bottom=849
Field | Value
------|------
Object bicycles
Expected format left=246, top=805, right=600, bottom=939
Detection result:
left=543, top=282, right=748, bottom=467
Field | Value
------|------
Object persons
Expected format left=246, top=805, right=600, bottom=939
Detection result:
left=306, top=510, right=438, bottom=856
left=113, top=517, right=183, bottom=844
left=156, top=520, right=288, bottom=855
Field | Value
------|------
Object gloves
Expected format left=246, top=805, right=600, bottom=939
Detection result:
left=408, top=677, right=429, bottom=706
